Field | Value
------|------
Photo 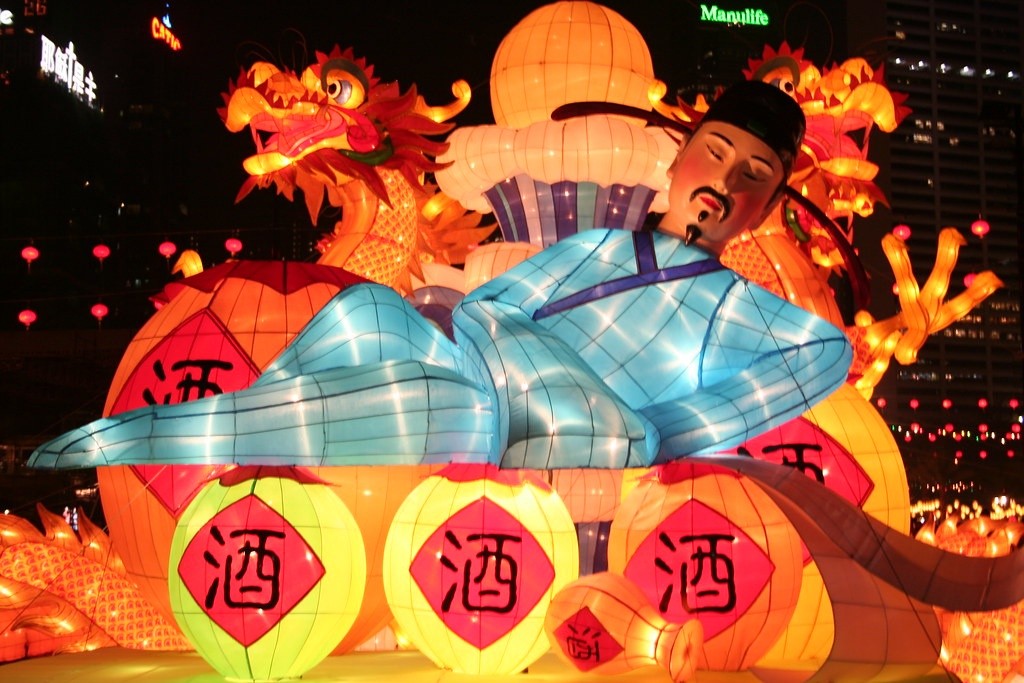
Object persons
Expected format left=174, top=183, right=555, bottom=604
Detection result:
left=653, top=79, right=807, bottom=264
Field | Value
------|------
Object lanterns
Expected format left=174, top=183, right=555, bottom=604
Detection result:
left=0, top=1, right=1023, bottom=683
